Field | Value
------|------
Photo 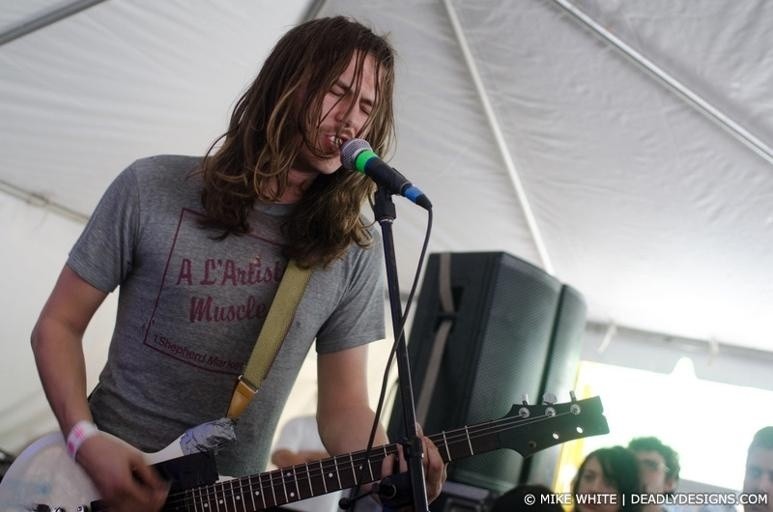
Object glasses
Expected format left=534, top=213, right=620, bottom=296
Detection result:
left=639, top=461, right=670, bottom=473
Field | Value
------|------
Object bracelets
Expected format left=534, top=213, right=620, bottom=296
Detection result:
left=63, top=417, right=96, bottom=461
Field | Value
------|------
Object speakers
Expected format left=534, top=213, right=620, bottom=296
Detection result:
left=524, top=284, right=587, bottom=490
left=385, top=251, right=565, bottom=493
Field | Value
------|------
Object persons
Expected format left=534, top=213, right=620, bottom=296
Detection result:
left=626, top=435, right=680, bottom=512
left=266, top=413, right=351, bottom=510
left=28, top=17, right=449, bottom=510
left=570, top=445, right=644, bottom=511
left=488, top=482, right=563, bottom=510
left=741, top=425, right=772, bottom=512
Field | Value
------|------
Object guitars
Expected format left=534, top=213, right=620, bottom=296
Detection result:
left=0, top=391, right=608, bottom=511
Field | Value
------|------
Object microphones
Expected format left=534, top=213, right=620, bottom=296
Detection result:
left=340, top=137, right=432, bottom=210
